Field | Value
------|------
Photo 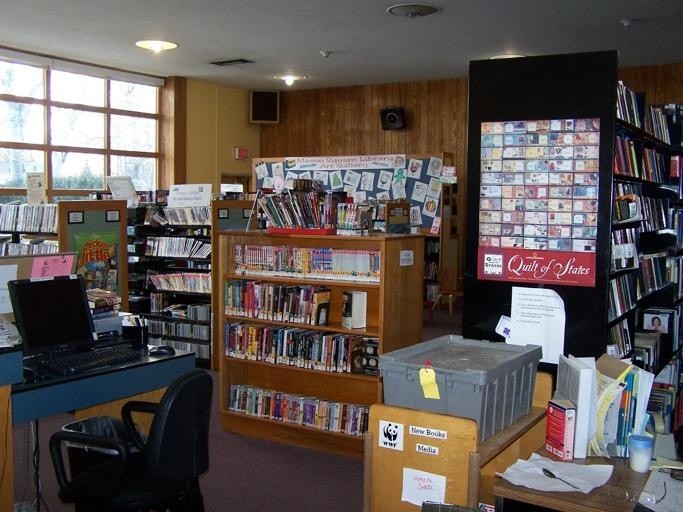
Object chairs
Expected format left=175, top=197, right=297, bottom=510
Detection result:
left=48, top=371, right=213, bottom=512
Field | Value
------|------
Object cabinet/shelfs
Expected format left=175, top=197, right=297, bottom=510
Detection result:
left=217, top=152, right=427, bottom=461
left=1, top=199, right=128, bottom=312
left=364, top=367, right=554, bottom=512
left=459, top=51, right=683, bottom=457
left=133, top=198, right=258, bottom=371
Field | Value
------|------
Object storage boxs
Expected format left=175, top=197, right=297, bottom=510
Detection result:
left=378, top=334, right=543, bottom=444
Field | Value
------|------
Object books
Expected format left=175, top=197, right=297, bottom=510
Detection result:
left=222, top=190, right=440, bottom=376
left=0, top=171, right=210, bottom=359
left=545, top=82, right=682, bottom=463
left=230, top=384, right=369, bottom=436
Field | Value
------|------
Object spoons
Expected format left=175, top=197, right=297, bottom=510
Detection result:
left=541, top=467, right=579, bottom=490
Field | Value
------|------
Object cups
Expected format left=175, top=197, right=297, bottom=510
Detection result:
left=628, top=434, right=654, bottom=473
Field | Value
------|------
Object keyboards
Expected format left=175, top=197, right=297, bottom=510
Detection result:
left=47, top=343, right=141, bottom=373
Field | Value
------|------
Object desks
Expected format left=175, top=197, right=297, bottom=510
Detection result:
left=1, top=313, right=196, bottom=512
left=490, top=444, right=656, bottom=511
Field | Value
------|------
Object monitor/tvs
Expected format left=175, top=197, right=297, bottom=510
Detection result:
left=6, top=274, right=99, bottom=357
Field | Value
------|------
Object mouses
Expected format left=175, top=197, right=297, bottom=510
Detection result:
left=150, top=345, right=176, bottom=356
